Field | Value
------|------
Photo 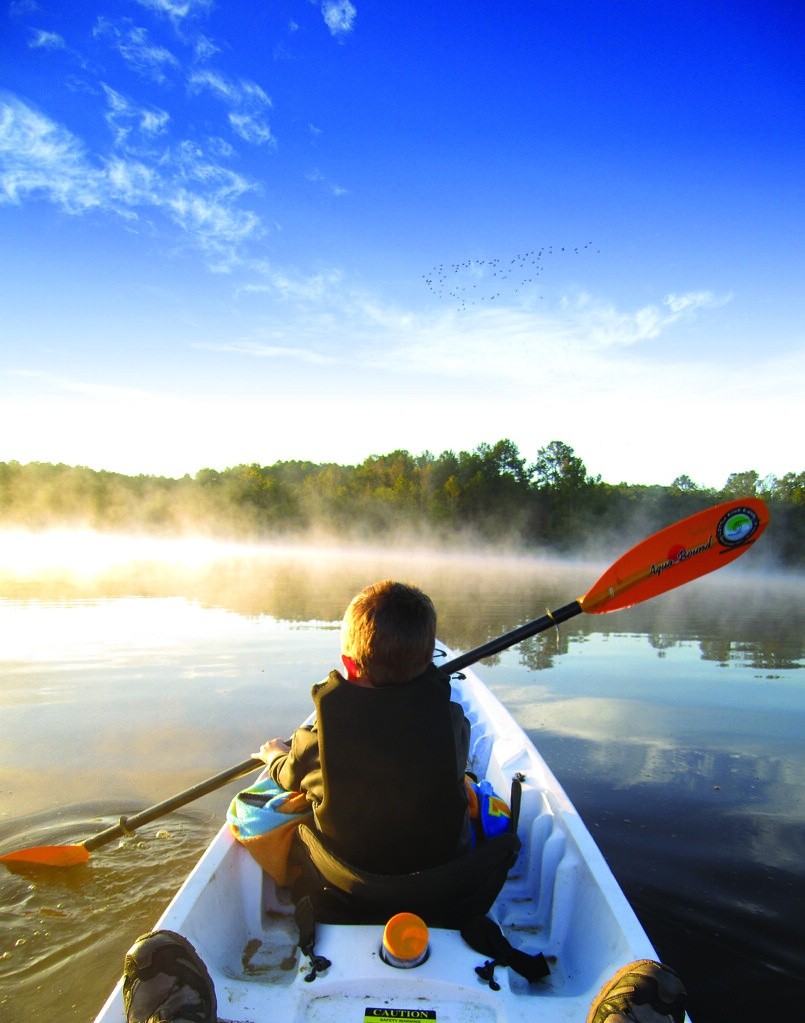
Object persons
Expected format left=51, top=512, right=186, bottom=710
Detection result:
left=250, top=579, right=471, bottom=924
left=121, top=929, right=689, bottom=1022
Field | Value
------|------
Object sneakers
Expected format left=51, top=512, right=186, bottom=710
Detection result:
left=587, top=959, right=687, bottom=1023
left=122, top=930, right=217, bottom=1023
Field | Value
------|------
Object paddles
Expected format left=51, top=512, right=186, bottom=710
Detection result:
left=1, top=491, right=772, bottom=870
